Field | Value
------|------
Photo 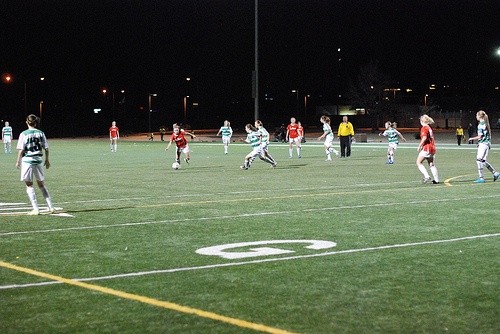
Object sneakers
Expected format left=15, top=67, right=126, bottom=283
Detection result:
left=240, top=166, right=248, bottom=170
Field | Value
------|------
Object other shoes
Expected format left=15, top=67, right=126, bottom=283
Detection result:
left=184, top=158, right=189, bottom=165
left=47, top=208, right=57, bottom=214
left=298, top=156, right=301, bottom=159
left=422, top=177, right=431, bottom=185
left=336, top=151, right=340, bottom=158
left=325, top=158, right=332, bottom=161
left=494, top=173, right=500, bottom=182
left=431, top=180, right=439, bottom=184
left=28, top=209, right=40, bottom=215
left=289, top=156, right=293, bottom=159
left=473, top=179, right=486, bottom=183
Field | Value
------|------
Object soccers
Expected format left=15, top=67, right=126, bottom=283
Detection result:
left=172, top=163, right=180, bottom=169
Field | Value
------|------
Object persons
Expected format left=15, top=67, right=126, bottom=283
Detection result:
left=318, top=115, right=339, bottom=162
left=159, top=127, right=165, bottom=141
left=165, top=125, right=196, bottom=170
left=16, top=114, right=58, bottom=215
left=239, top=120, right=277, bottom=170
left=378, top=121, right=406, bottom=165
left=455, top=125, right=463, bottom=146
left=467, top=123, right=474, bottom=144
left=462, top=133, right=467, bottom=144
left=217, top=120, right=233, bottom=154
left=189, top=130, right=194, bottom=141
left=414, top=115, right=440, bottom=184
left=149, top=132, right=154, bottom=142
left=337, top=116, right=355, bottom=158
left=109, top=121, right=119, bottom=152
left=1, top=122, right=13, bottom=153
left=278, top=118, right=306, bottom=158
left=468, top=110, right=500, bottom=184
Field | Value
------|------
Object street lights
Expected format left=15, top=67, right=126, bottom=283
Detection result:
left=305, top=94, right=310, bottom=124
left=424, top=94, right=428, bottom=106
left=371, top=86, right=381, bottom=127
left=184, top=95, right=189, bottom=129
left=292, top=89, right=299, bottom=123
left=337, top=95, right=341, bottom=125
left=149, top=93, right=157, bottom=132
